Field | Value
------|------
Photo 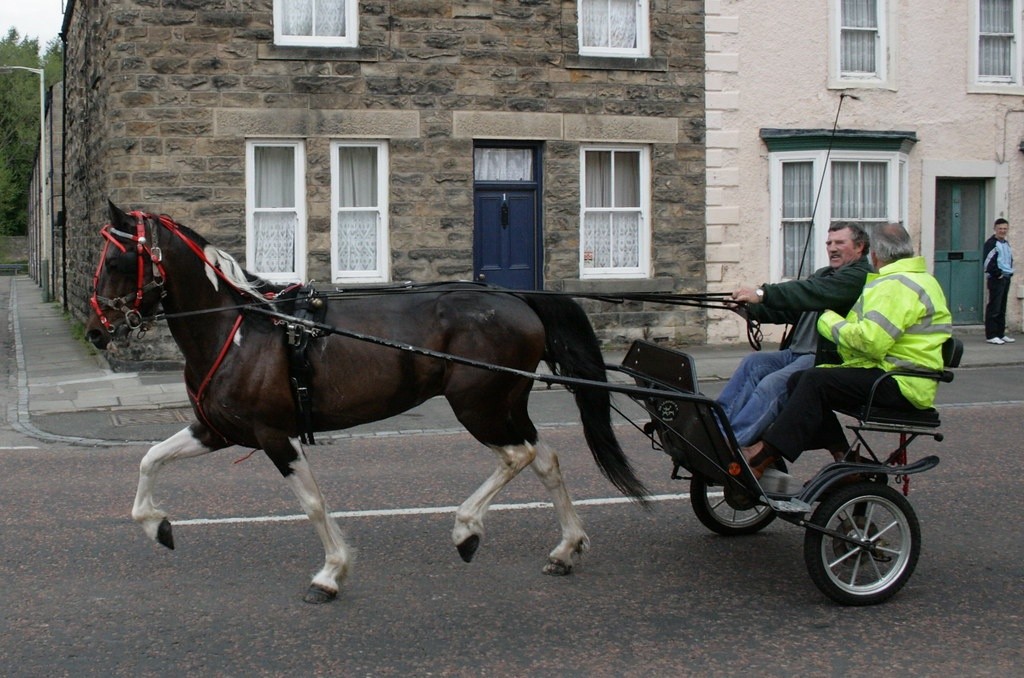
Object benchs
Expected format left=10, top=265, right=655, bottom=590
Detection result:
left=834, top=336, right=963, bottom=427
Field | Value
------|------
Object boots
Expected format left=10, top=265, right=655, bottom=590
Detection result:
left=740, top=441, right=775, bottom=479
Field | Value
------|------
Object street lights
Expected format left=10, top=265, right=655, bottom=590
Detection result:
left=0, top=66, right=50, bottom=305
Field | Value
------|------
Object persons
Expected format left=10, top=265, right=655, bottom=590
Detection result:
left=712, top=221, right=874, bottom=459
left=739, top=221, right=953, bottom=480
left=983, top=218, right=1016, bottom=345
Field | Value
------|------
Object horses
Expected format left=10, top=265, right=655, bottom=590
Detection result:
left=86, top=200, right=657, bottom=604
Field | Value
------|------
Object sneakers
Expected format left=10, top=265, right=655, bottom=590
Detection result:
left=1001, top=336, right=1015, bottom=342
left=986, top=336, right=1005, bottom=344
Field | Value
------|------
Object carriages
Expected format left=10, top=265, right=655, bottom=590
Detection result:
left=83, top=198, right=964, bottom=609
